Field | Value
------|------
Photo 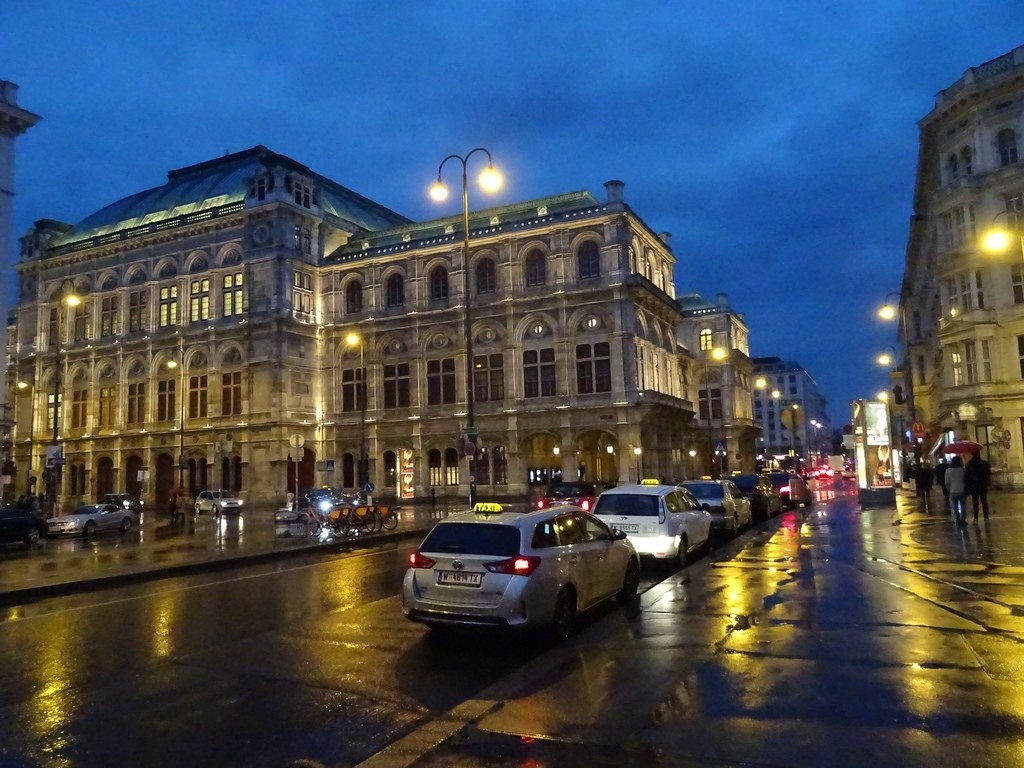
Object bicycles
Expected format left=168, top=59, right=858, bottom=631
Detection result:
left=303, top=494, right=399, bottom=539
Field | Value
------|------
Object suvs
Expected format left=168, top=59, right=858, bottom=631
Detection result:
left=195, top=489, right=245, bottom=516
left=103, top=493, right=145, bottom=515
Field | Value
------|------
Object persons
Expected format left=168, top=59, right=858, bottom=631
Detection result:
left=917, top=462, right=934, bottom=504
left=934, top=457, right=951, bottom=508
left=964, top=450, right=993, bottom=524
left=944, top=456, right=968, bottom=528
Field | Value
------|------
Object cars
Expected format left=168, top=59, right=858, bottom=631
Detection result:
left=400, top=454, right=856, bottom=635
left=0, top=506, right=49, bottom=548
left=300, top=487, right=342, bottom=507
left=46, top=503, right=137, bottom=537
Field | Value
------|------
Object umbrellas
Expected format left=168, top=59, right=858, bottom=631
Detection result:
left=946, top=439, right=985, bottom=464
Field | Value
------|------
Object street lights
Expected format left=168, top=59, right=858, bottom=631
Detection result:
left=876, top=345, right=909, bottom=485
left=168, top=347, right=190, bottom=489
left=47, top=276, right=82, bottom=513
left=427, top=146, right=507, bottom=512
left=344, top=321, right=369, bottom=491
left=703, top=341, right=827, bottom=480
left=881, top=291, right=931, bottom=511
